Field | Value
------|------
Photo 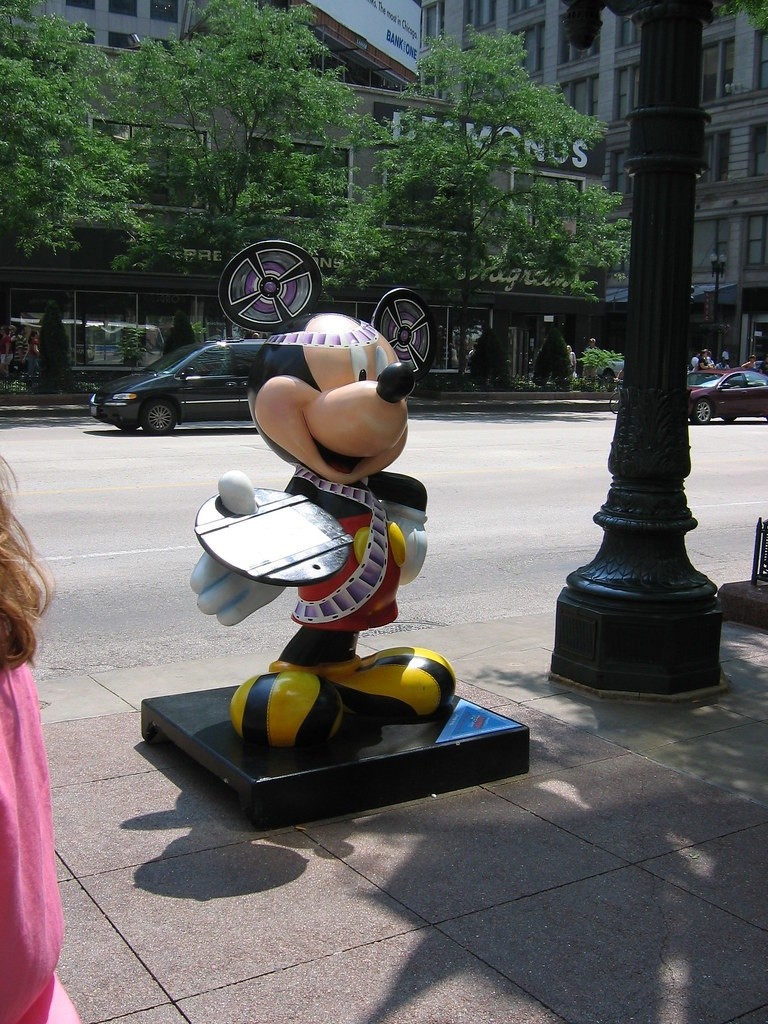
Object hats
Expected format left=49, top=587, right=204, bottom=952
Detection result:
left=9, top=325, right=17, bottom=329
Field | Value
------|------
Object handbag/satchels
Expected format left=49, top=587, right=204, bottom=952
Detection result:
left=9, top=359, right=23, bottom=372
left=22, top=358, right=28, bottom=365
left=2, top=353, right=14, bottom=377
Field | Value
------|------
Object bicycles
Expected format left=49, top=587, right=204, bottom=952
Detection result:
left=609, top=379, right=624, bottom=414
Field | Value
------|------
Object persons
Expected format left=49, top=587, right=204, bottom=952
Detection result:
left=721, top=346, right=730, bottom=361
left=567, top=345, right=576, bottom=378
left=0, top=324, right=39, bottom=378
left=760, top=356, right=768, bottom=375
left=688, top=349, right=730, bottom=377
left=588, top=338, right=597, bottom=350
left=741, top=354, right=756, bottom=370
left=0, top=455, right=64, bottom=1024
left=617, top=368, right=624, bottom=378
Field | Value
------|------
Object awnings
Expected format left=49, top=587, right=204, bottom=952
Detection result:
left=604, top=283, right=737, bottom=302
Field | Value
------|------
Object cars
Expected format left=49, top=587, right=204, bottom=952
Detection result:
left=90, top=336, right=267, bottom=437
left=688, top=369, right=768, bottom=425
left=9, top=317, right=165, bottom=384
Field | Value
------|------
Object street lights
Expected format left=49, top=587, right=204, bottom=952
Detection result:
left=709, top=247, right=730, bottom=366
